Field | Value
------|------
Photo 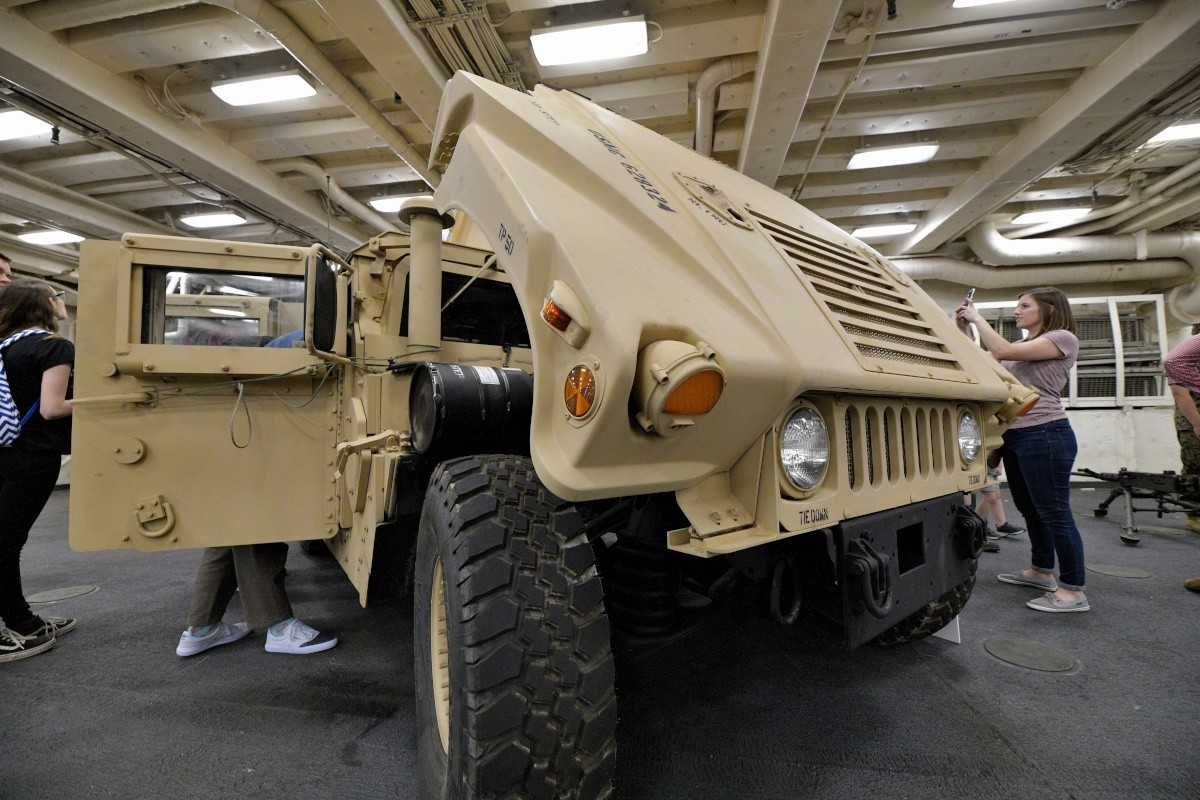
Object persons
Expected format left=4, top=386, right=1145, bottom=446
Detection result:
left=953, top=287, right=1092, bottom=614
left=1162, top=323, right=1200, bottom=594
left=0, top=252, right=77, bottom=662
left=175, top=327, right=338, bottom=657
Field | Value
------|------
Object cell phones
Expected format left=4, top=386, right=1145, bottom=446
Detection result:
left=960, top=288, right=976, bottom=321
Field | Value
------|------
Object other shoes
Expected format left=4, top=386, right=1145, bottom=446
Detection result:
left=1184, top=516, right=1200, bottom=532
left=982, top=540, right=1000, bottom=552
left=1183, top=576, right=1200, bottom=591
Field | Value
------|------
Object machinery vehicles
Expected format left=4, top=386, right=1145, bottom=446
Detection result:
left=68, top=69, right=1040, bottom=800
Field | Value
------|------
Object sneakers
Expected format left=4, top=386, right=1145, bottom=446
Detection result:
left=998, top=570, right=1058, bottom=592
left=24, top=614, right=78, bottom=638
left=987, top=527, right=1000, bottom=540
left=176, top=621, right=252, bottom=657
left=0, top=617, right=56, bottom=662
left=264, top=619, right=338, bottom=654
left=994, top=522, right=1026, bottom=537
left=1026, top=592, right=1090, bottom=612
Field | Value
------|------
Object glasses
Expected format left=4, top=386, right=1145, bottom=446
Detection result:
left=53, top=290, right=68, bottom=301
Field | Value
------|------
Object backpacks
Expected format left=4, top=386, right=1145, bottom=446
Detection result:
left=0, top=328, right=53, bottom=448
